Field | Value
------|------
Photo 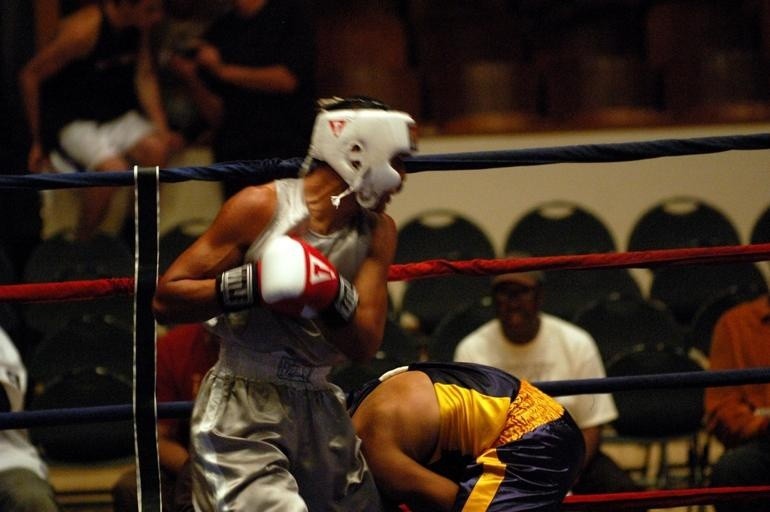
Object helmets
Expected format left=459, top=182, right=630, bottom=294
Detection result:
left=310, top=109, right=419, bottom=221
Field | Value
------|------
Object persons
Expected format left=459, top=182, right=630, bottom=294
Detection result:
left=154, top=97, right=417, bottom=511
left=110, top=317, right=218, bottom=512
left=392, top=0, right=770, bottom=129
left=330, top=253, right=651, bottom=512
left=1, top=330, right=59, bottom=512
left=702, top=293, right=769, bottom=512
left=23, top=2, right=318, bottom=279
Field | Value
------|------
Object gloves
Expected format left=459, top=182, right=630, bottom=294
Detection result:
left=219, top=262, right=317, bottom=319
left=258, top=235, right=359, bottom=323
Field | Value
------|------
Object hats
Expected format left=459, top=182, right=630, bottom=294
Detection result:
left=490, top=257, right=544, bottom=288
